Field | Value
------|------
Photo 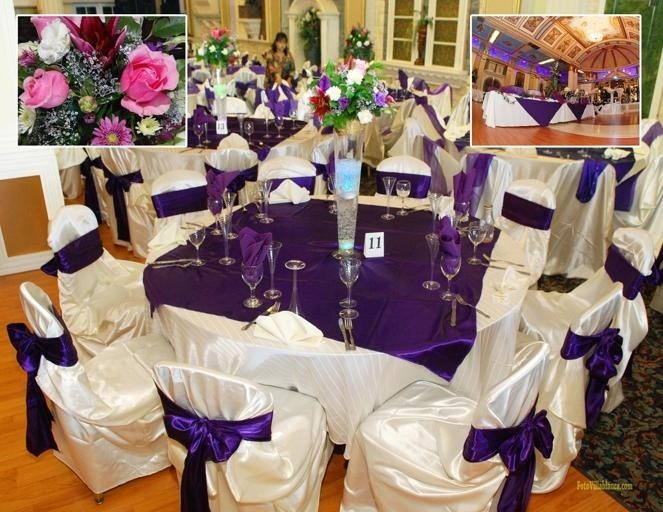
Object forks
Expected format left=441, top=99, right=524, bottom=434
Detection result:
left=344, top=318, right=356, bottom=350
left=269, top=301, right=280, bottom=315
left=482, top=253, right=525, bottom=267
left=151, top=262, right=192, bottom=269
left=455, top=294, right=490, bottom=319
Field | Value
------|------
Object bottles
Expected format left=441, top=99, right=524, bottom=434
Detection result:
left=480, top=204, right=495, bottom=244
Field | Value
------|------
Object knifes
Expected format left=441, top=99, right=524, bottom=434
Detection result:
left=450, top=298, right=456, bottom=326
left=152, top=259, right=191, bottom=264
left=338, top=318, right=350, bottom=350
left=479, top=263, right=530, bottom=276
left=240, top=304, right=274, bottom=331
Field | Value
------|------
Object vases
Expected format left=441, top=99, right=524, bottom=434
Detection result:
left=302, top=39, right=321, bottom=70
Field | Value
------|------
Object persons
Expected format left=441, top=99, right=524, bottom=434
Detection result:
left=265, top=32, right=296, bottom=90
left=599, top=83, right=640, bottom=104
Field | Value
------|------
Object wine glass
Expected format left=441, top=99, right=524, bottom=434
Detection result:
left=380, top=177, right=397, bottom=220
left=249, top=178, right=274, bottom=225
left=395, top=180, right=411, bottom=216
left=449, top=202, right=467, bottom=236
left=421, top=233, right=440, bottom=291
left=206, top=197, right=223, bottom=236
left=335, top=256, right=361, bottom=319
left=325, top=173, right=337, bottom=215
left=240, top=261, right=264, bottom=309
left=388, top=87, right=414, bottom=102
left=440, top=255, right=461, bottom=301
left=217, top=212, right=236, bottom=266
left=428, top=192, right=442, bottom=221
left=262, top=241, right=282, bottom=300
left=188, top=106, right=297, bottom=149
left=222, top=191, right=238, bottom=239
left=187, top=222, right=206, bottom=267
left=465, top=221, right=486, bottom=265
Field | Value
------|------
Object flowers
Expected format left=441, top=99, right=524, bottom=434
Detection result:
left=344, top=20, right=376, bottom=62
left=19, top=15, right=185, bottom=147
left=296, top=7, right=321, bottom=43
left=193, top=19, right=242, bottom=70
